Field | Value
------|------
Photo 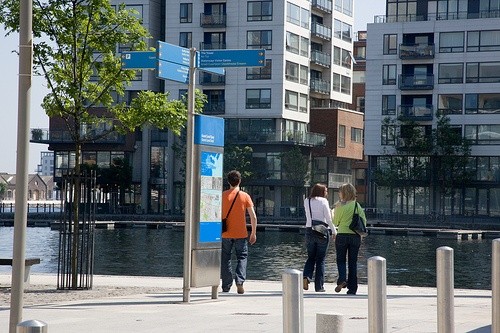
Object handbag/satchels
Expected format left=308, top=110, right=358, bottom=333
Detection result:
left=311, top=220, right=329, bottom=239
left=349, top=213, right=367, bottom=235
left=222, top=218, right=227, bottom=233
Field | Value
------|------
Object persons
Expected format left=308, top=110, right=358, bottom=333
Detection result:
left=221, top=171, right=257, bottom=294
left=302, top=184, right=337, bottom=292
left=332, top=183, right=366, bottom=295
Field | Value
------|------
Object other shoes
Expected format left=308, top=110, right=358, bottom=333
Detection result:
left=335, top=281, right=346, bottom=292
left=237, top=284, right=244, bottom=294
left=303, top=277, right=309, bottom=290
left=316, top=289, right=325, bottom=292
